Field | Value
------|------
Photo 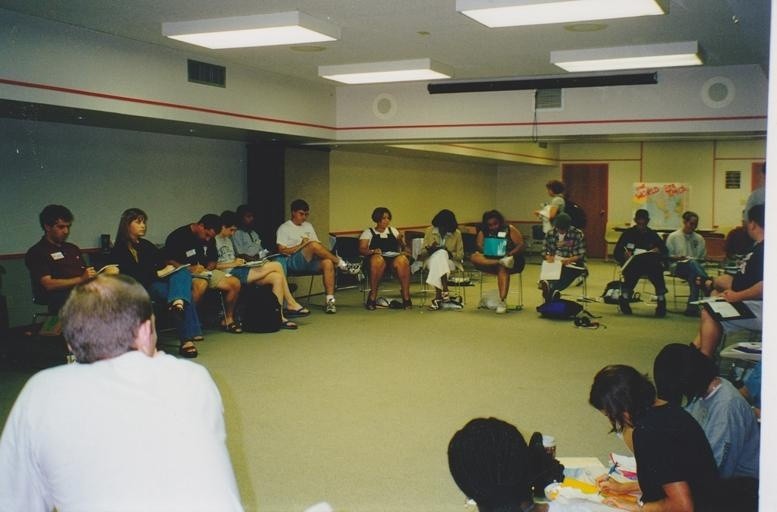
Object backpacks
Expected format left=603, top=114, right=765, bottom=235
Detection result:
left=552, top=196, right=586, bottom=229
left=242, top=286, right=281, bottom=333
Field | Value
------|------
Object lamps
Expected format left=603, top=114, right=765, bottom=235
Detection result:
left=317, top=59, right=455, bottom=85
left=548, top=41, right=707, bottom=73
left=160, top=11, right=341, bottom=49
left=456, top=0, right=668, bottom=30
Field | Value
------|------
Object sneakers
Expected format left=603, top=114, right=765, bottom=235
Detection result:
left=537, top=280, right=701, bottom=318
left=325, top=298, right=337, bottom=314
left=501, top=255, right=515, bottom=270
left=496, top=301, right=507, bottom=314
left=338, top=263, right=360, bottom=275
left=528, top=432, right=565, bottom=498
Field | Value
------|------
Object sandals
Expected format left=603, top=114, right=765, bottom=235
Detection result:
left=400, top=289, right=413, bottom=310
left=168, top=300, right=243, bottom=356
left=428, top=290, right=449, bottom=311
left=367, top=289, right=378, bottom=310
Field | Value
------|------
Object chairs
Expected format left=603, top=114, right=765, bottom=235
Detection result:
left=334, top=231, right=524, bottom=310
left=271, top=236, right=328, bottom=316
left=30, top=295, right=66, bottom=368
left=195, top=287, right=229, bottom=335
left=618, top=263, right=659, bottom=310
left=96, top=255, right=184, bottom=351
left=661, top=233, right=704, bottom=309
left=715, top=324, right=762, bottom=366
left=575, top=264, right=588, bottom=300
left=719, top=341, right=761, bottom=390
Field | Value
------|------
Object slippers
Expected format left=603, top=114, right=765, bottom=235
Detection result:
left=281, top=308, right=310, bottom=329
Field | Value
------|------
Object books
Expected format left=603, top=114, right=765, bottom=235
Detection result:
left=96, top=264, right=118, bottom=275
left=241, top=258, right=268, bottom=267
left=158, top=263, right=190, bottom=278
left=294, top=239, right=322, bottom=254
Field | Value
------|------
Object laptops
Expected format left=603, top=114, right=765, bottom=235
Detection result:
left=482, top=237, right=509, bottom=257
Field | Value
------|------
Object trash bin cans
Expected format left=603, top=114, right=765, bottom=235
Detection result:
left=719, top=341, right=761, bottom=408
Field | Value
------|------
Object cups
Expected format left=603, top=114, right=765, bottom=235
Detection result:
left=540, top=436, right=556, bottom=462
left=100, top=233, right=111, bottom=252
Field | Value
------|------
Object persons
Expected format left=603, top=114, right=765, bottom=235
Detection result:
left=448, top=414, right=631, bottom=512
left=276, top=199, right=361, bottom=314
left=417, top=209, right=465, bottom=310
left=108, top=207, right=201, bottom=358
left=0, top=273, right=245, bottom=511
left=358, top=207, right=412, bottom=310
left=215, top=211, right=310, bottom=328
left=469, top=210, right=524, bottom=314
left=540, top=212, right=585, bottom=302
left=166, top=213, right=243, bottom=342
left=528, top=364, right=721, bottom=512
left=533, top=180, right=584, bottom=288
left=230, top=205, right=288, bottom=318
left=26, top=205, right=96, bottom=314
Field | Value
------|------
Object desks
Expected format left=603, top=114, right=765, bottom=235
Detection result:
left=613, top=227, right=724, bottom=262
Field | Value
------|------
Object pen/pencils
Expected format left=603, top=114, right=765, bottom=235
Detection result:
left=688, top=298, right=727, bottom=305
left=80, top=266, right=86, bottom=269
left=595, top=461, right=618, bottom=498
left=624, top=246, right=634, bottom=262
left=197, top=261, right=200, bottom=268
